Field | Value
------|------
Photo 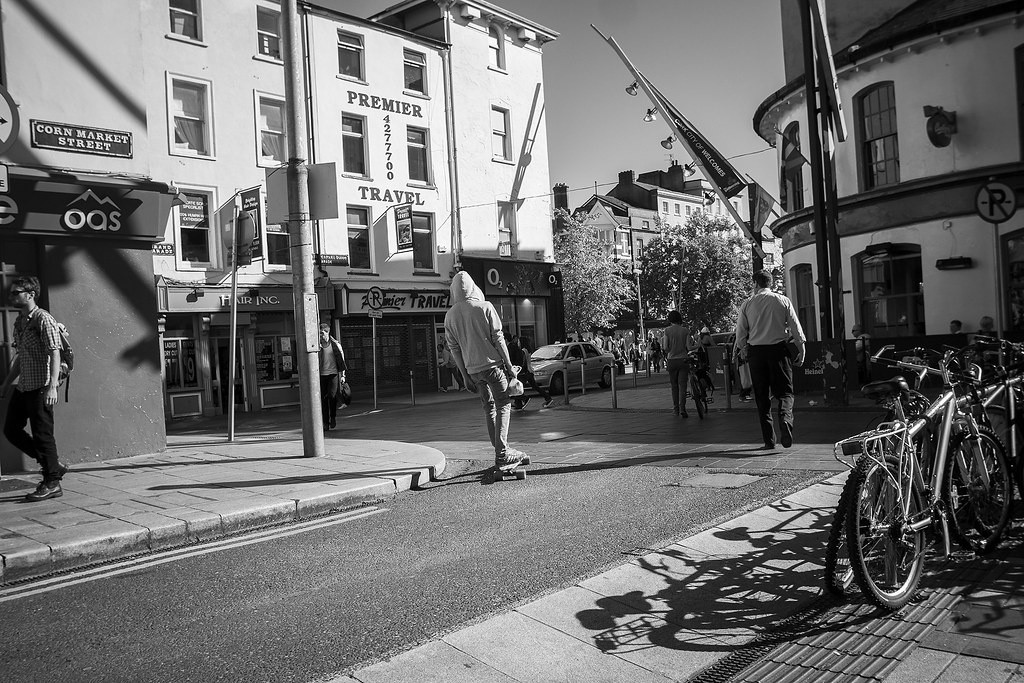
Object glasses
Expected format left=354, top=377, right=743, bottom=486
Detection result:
left=851, top=329, right=860, bottom=333
left=8, top=291, right=30, bottom=296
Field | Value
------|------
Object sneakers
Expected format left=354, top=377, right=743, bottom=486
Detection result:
left=544, top=399, right=553, bottom=408
left=338, top=402, right=347, bottom=410
left=494, top=446, right=526, bottom=468
left=41, top=462, right=69, bottom=481
left=521, top=398, right=531, bottom=409
left=26, top=479, right=62, bottom=501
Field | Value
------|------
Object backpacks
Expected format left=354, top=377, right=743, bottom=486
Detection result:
left=18, top=308, right=73, bottom=380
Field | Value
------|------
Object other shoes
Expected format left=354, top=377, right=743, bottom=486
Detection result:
left=780, top=420, right=793, bottom=447
left=745, top=395, right=753, bottom=399
left=673, top=410, right=679, bottom=416
left=324, top=423, right=329, bottom=430
left=680, top=410, right=688, bottom=418
left=739, top=398, right=750, bottom=402
left=760, top=440, right=774, bottom=449
left=330, top=419, right=336, bottom=428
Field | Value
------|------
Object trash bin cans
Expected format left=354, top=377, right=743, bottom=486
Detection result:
left=785, top=339, right=857, bottom=392
left=706, top=331, right=737, bottom=389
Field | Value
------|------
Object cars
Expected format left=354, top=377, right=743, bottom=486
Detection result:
left=525, top=341, right=618, bottom=396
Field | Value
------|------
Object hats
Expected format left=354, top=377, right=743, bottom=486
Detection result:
left=701, top=327, right=710, bottom=333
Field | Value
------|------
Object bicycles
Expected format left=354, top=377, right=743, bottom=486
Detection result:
left=686, top=346, right=715, bottom=421
left=823, top=336, right=1024, bottom=612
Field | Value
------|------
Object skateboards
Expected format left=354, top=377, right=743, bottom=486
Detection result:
left=494, top=451, right=531, bottom=480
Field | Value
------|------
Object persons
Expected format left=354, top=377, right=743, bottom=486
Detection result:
left=337, top=387, right=348, bottom=409
left=850, top=324, right=870, bottom=363
left=732, top=341, right=754, bottom=403
left=736, top=268, right=808, bottom=448
left=629, top=332, right=667, bottom=373
left=949, top=320, right=969, bottom=346
left=0, top=276, right=70, bottom=502
left=686, top=326, right=717, bottom=374
left=664, top=310, right=701, bottom=418
left=970, top=316, right=996, bottom=345
left=436, top=344, right=467, bottom=392
left=318, top=323, right=347, bottom=431
left=577, top=334, right=630, bottom=365
left=444, top=271, right=528, bottom=467
left=502, top=331, right=554, bottom=412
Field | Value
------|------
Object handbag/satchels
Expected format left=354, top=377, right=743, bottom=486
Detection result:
left=340, top=382, right=351, bottom=405
left=739, top=361, right=753, bottom=389
left=507, top=365, right=524, bottom=397
